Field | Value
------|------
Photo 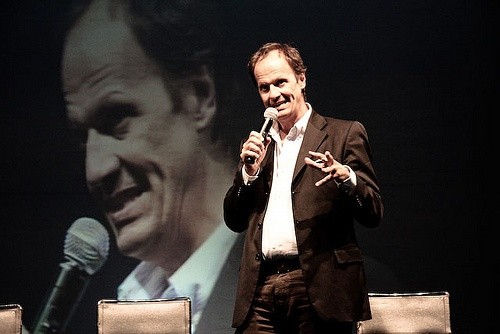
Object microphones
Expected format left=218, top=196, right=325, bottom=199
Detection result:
left=30, top=217, right=109, bottom=334
left=244, top=107, right=278, bottom=164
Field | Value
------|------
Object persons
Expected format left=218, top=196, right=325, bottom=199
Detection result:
left=62, top=0, right=249, bottom=334
left=223, top=42, right=383, bottom=334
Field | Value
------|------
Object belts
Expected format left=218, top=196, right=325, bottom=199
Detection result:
left=260, top=254, right=302, bottom=275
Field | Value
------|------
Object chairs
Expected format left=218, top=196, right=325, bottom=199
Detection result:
left=96, top=297, right=193, bottom=334
left=355, top=292, right=454, bottom=334
